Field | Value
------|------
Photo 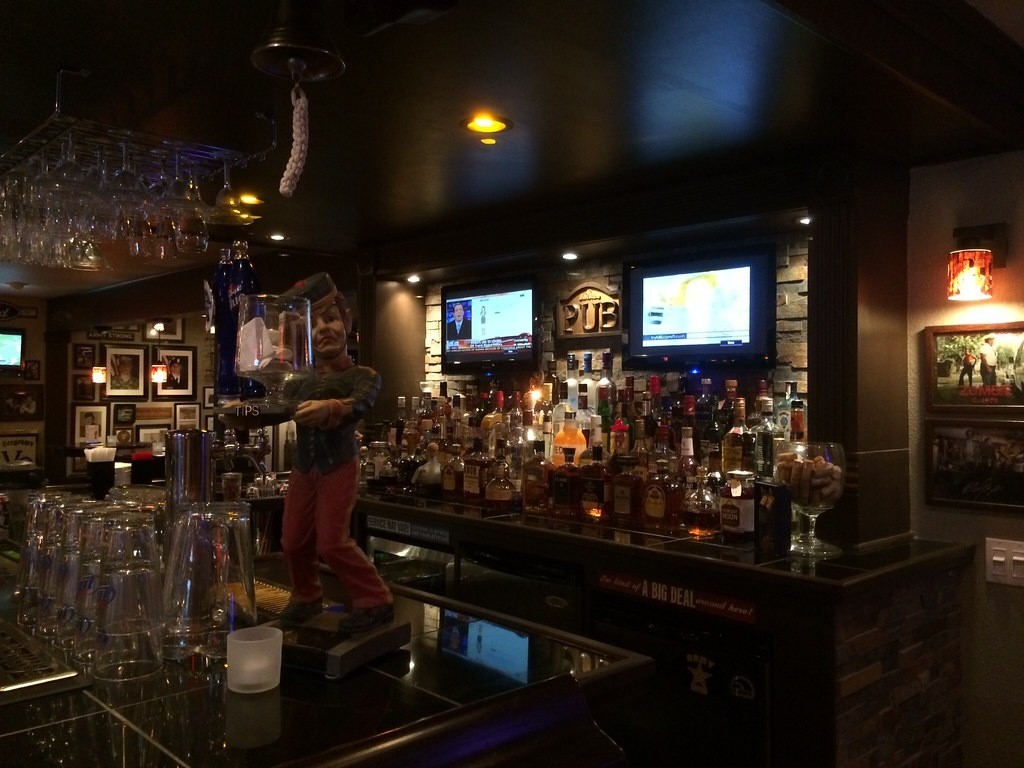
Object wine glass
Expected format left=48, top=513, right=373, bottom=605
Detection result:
left=773, top=442, right=845, bottom=558
left=0, top=135, right=242, bottom=264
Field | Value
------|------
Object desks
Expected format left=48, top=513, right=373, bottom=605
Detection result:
left=0, top=479, right=977, bottom=768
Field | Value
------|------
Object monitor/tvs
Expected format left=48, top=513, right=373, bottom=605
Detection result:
left=621, top=243, right=779, bottom=371
left=0, top=328, right=27, bottom=375
left=436, top=607, right=531, bottom=689
left=441, top=276, right=540, bottom=375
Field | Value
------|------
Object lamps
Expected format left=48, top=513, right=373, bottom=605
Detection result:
left=947, top=223, right=1009, bottom=301
left=151, top=329, right=168, bottom=382
left=92, top=319, right=107, bottom=383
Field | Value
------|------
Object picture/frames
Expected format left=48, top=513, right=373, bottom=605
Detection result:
left=924, top=323, right=1024, bottom=513
left=0, top=384, right=44, bottom=421
left=71, top=319, right=215, bottom=447
left=25, top=360, right=40, bottom=380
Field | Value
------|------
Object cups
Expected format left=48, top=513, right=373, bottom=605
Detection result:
left=131, top=460, right=154, bottom=483
left=222, top=473, right=241, bottom=500
left=87, top=461, right=114, bottom=496
left=227, top=627, right=282, bottom=693
left=16, top=483, right=163, bottom=681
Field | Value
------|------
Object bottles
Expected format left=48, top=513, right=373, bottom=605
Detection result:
left=358, top=355, right=806, bottom=554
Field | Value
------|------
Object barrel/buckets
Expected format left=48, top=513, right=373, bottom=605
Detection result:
left=164, top=429, right=215, bottom=512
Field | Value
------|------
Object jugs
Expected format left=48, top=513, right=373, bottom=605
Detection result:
left=162, top=504, right=255, bottom=661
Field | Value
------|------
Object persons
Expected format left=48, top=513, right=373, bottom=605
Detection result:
left=76, top=347, right=193, bottom=451
left=447, top=303, right=472, bottom=339
left=932, top=430, right=1024, bottom=485
left=956, top=336, right=999, bottom=399
left=220, top=272, right=398, bottom=635
left=479, top=306, right=488, bottom=336
